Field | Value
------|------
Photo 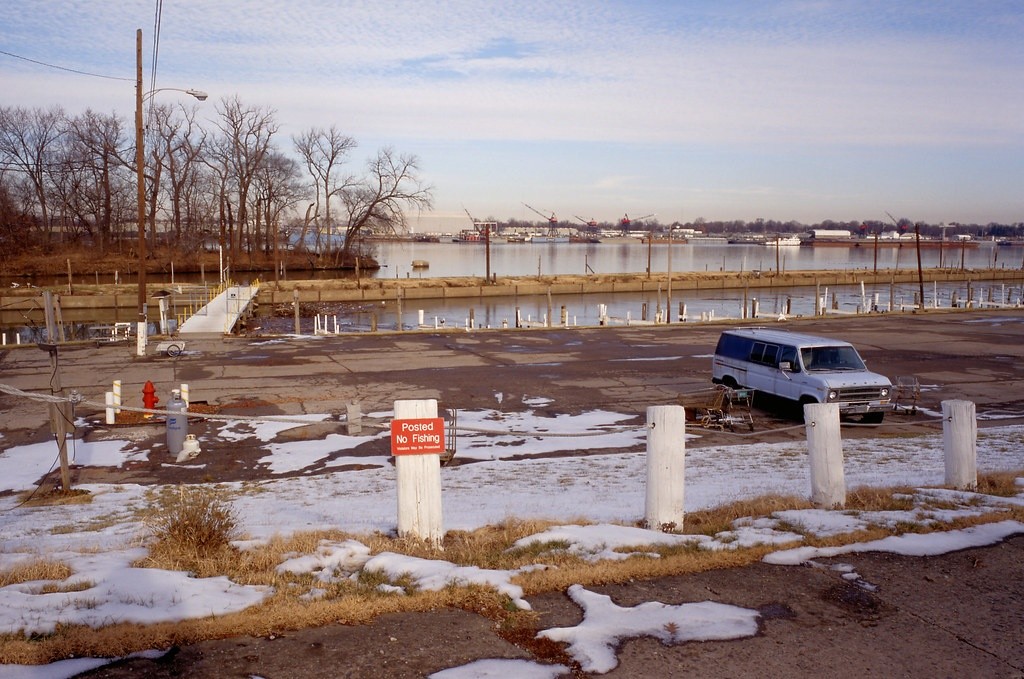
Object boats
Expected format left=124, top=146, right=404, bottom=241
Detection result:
left=997, top=236, right=1014, bottom=247
left=569, top=232, right=601, bottom=243
left=763, top=234, right=802, bottom=246
left=419, top=235, right=440, bottom=243
left=452, top=229, right=491, bottom=243
left=729, top=235, right=767, bottom=244
left=507, top=233, right=533, bottom=242
left=411, top=260, right=429, bottom=267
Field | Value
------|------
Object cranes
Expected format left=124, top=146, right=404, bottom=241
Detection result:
left=846, top=222, right=868, bottom=239
left=465, top=209, right=487, bottom=241
left=619, top=213, right=655, bottom=238
left=576, top=214, right=598, bottom=238
left=523, top=202, right=558, bottom=236
left=885, top=210, right=908, bottom=234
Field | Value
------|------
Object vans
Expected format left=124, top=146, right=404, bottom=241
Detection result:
left=712, top=328, right=893, bottom=427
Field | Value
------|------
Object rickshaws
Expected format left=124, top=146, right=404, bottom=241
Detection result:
left=149, top=341, right=186, bottom=357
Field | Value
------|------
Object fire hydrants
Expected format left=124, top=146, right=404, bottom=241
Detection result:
left=140, top=378, right=159, bottom=419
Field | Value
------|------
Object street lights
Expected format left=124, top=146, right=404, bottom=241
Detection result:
left=136, top=27, right=209, bottom=356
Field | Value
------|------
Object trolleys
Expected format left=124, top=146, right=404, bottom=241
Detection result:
left=436, top=404, right=459, bottom=465
left=699, top=384, right=756, bottom=432
left=894, top=375, right=921, bottom=415
